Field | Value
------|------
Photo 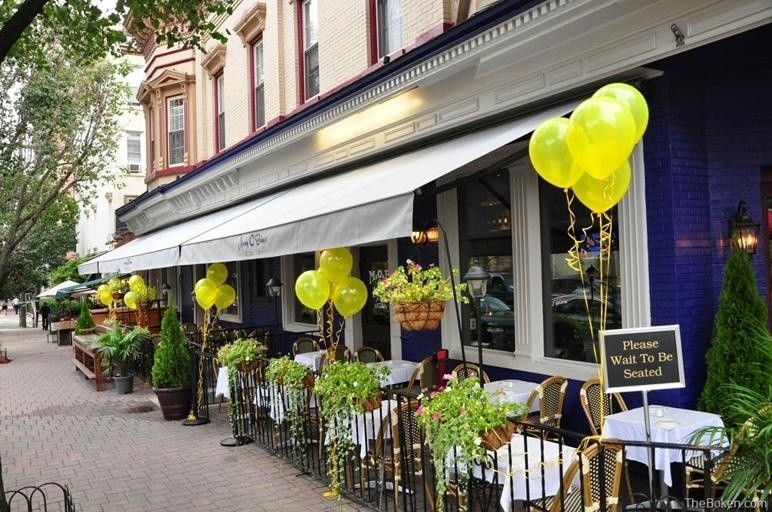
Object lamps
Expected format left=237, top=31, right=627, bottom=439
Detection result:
left=410, top=217, right=473, bottom=385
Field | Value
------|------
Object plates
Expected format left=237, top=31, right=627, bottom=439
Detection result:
left=654, top=420, right=681, bottom=429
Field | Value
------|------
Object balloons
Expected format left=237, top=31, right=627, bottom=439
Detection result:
left=293, top=249, right=368, bottom=319
left=527, top=82, right=651, bottom=215
left=96, top=273, right=156, bottom=309
left=193, top=262, right=236, bottom=310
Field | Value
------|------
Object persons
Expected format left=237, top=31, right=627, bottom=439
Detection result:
left=12, top=296, right=20, bottom=313
left=3, top=298, right=9, bottom=314
left=481, top=274, right=510, bottom=312
left=40, top=302, right=50, bottom=331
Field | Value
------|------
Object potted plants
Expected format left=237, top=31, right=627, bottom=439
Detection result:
left=90, top=319, right=156, bottom=397
left=151, top=308, right=192, bottom=421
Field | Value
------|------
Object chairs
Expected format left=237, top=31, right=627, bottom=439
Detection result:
left=152, top=322, right=772, bottom=512
left=45, top=313, right=78, bottom=346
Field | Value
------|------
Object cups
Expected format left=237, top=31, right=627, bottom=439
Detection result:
left=655, top=408, right=663, bottom=417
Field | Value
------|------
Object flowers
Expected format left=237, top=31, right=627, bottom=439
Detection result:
left=371, top=255, right=468, bottom=307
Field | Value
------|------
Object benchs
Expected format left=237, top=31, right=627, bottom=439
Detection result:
left=72, top=322, right=128, bottom=379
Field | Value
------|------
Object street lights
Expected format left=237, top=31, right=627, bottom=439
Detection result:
left=462, top=263, right=489, bottom=393
left=161, top=281, right=171, bottom=309
left=264, top=277, right=284, bottom=355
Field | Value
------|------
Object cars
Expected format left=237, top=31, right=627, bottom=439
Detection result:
left=462, top=263, right=621, bottom=363
left=299, top=307, right=316, bottom=321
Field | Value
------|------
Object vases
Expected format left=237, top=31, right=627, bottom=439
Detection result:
left=391, top=295, right=445, bottom=333
